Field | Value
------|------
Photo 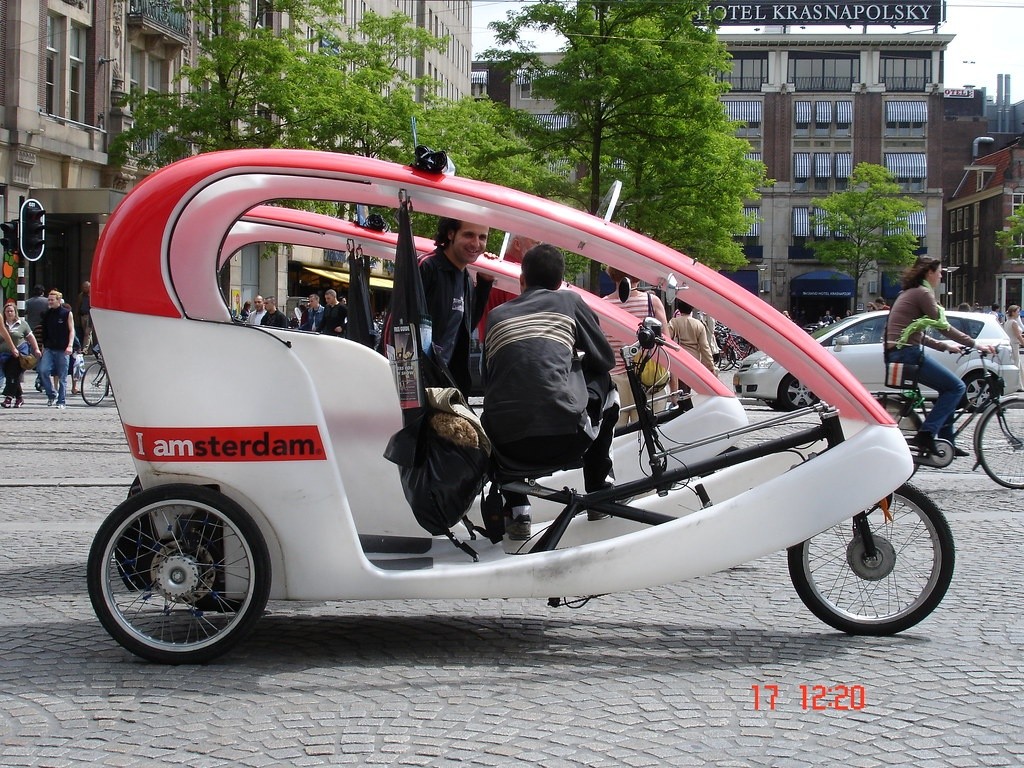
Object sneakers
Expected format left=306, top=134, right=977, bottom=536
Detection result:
left=505, top=508, right=531, bottom=540
left=587, top=496, right=635, bottom=521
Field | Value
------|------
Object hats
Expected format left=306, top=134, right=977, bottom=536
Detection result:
left=876, top=297, right=885, bottom=305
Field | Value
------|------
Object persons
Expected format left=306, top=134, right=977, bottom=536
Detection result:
left=415, top=216, right=495, bottom=402
left=818, top=310, right=853, bottom=322
left=955, top=300, right=1024, bottom=393
left=596, top=266, right=719, bottom=424
left=866, top=296, right=891, bottom=311
left=480, top=233, right=635, bottom=541
left=38, top=290, right=76, bottom=410
left=856, top=322, right=873, bottom=343
left=237, top=289, right=389, bottom=339
left=54, top=336, right=83, bottom=394
left=0, top=313, right=19, bottom=357
left=884, top=253, right=996, bottom=457
left=79, top=279, right=92, bottom=356
left=24, top=284, right=49, bottom=331
left=0, top=302, right=42, bottom=408
left=783, top=311, right=791, bottom=319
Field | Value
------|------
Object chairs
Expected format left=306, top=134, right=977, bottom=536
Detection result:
left=426, top=339, right=583, bottom=504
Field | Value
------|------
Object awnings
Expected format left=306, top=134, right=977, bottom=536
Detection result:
left=549, top=158, right=570, bottom=178
left=516, top=68, right=569, bottom=85
left=716, top=100, right=762, bottom=238
left=789, top=271, right=858, bottom=298
left=471, top=71, right=487, bottom=85
left=530, top=115, right=570, bottom=131
left=794, top=100, right=852, bottom=237
left=880, top=269, right=905, bottom=299
left=718, top=270, right=758, bottom=296
left=884, top=100, right=928, bottom=238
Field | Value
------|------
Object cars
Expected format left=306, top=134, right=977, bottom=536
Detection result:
left=731, top=307, right=1020, bottom=414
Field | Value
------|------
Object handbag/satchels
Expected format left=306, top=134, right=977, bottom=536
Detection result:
left=633, top=351, right=671, bottom=392
left=884, top=363, right=920, bottom=389
left=383, top=343, right=493, bottom=536
left=19, top=353, right=37, bottom=369
left=72, top=351, right=86, bottom=381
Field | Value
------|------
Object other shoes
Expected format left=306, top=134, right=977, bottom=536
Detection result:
left=954, top=446, right=970, bottom=456
left=14, top=397, right=24, bottom=408
left=0, top=397, right=12, bottom=408
left=1016, top=389, right=1024, bottom=392
left=914, top=431, right=942, bottom=458
left=47, top=399, right=54, bottom=405
left=71, top=389, right=82, bottom=395
left=56, top=405, right=65, bottom=409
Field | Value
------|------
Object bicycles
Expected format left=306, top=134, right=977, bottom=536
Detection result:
left=868, top=345, right=1024, bottom=490
left=712, top=319, right=759, bottom=372
left=81, top=349, right=110, bottom=406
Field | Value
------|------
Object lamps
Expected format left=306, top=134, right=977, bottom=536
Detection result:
left=756, top=265, right=768, bottom=271
left=26, top=128, right=44, bottom=135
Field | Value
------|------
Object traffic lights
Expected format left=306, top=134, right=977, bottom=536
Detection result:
left=20, top=198, right=48, bottom=261
left=0, top=221, right=19, bottom=251
